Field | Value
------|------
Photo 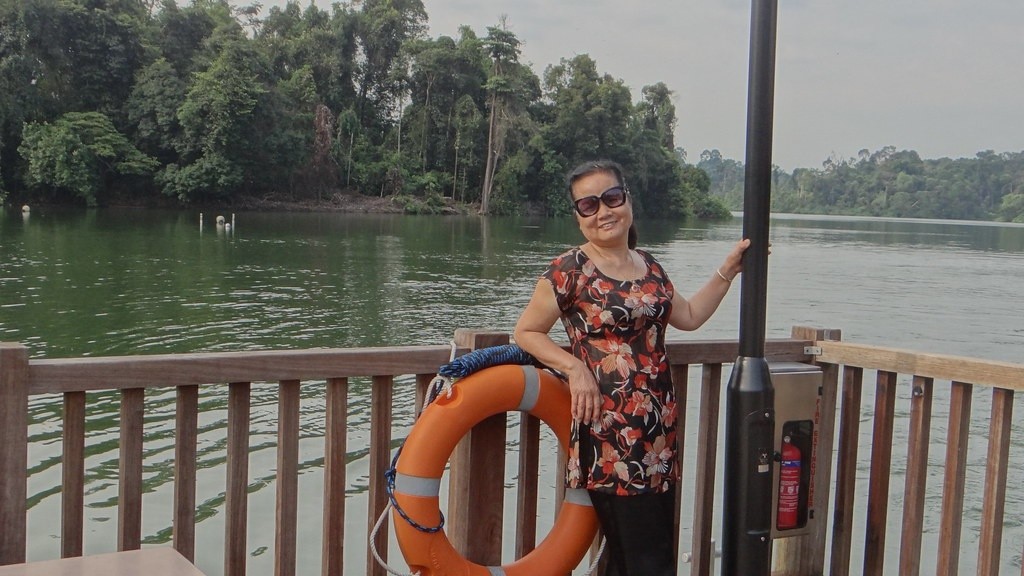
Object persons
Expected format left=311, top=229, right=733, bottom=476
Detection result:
left=513, top=159, right=773, bottom=576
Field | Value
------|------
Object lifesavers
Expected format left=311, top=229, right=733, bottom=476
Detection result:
left=392, top=363, right=599, bottom=575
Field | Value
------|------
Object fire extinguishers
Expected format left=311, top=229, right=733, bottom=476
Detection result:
left=777, top=424, right=802, bottom=529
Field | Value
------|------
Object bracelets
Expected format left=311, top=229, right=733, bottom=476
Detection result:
left=716, top=267, right=734, bottom=282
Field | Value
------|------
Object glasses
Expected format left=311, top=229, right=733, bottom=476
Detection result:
left=573, top=186, right=629, bottom=218
